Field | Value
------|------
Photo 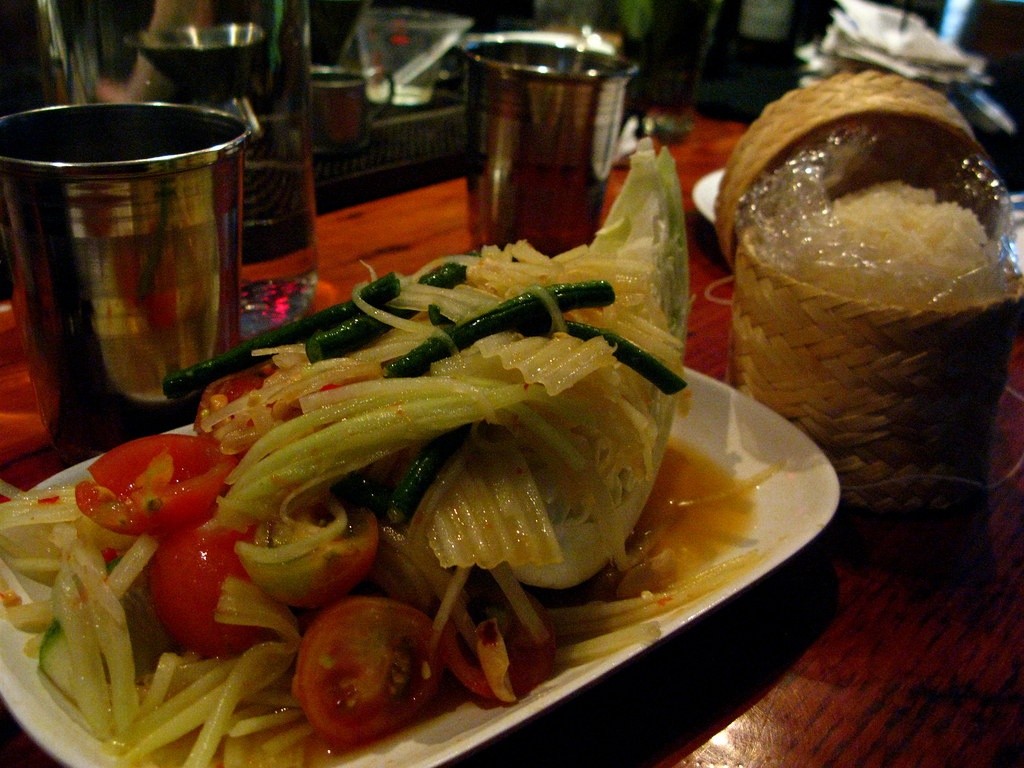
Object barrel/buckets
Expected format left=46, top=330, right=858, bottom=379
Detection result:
left=0, top=98, right=262, bottom=470
left=454, top=32, right=632, bottom=262
left=1, top=1, right=318, bottom=342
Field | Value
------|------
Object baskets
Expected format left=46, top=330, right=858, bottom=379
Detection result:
left=731, top=240, right=1023, bottom=513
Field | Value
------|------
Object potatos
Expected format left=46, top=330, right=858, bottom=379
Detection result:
left=1, top=137, right=787, bottom=767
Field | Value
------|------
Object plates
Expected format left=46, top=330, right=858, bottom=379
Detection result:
left=1, top=362, right=842, bottom=767
left=692, top=166, right=724, bottom=223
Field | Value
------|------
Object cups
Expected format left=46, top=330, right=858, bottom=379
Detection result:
left=311, top=66, right=395, bottom=152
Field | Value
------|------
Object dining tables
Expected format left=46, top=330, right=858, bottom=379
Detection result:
left=3, top=118, right=1024, bottom=768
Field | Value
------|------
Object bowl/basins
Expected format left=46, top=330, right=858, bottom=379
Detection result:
left=354, top=10, right=473, bottom=107
